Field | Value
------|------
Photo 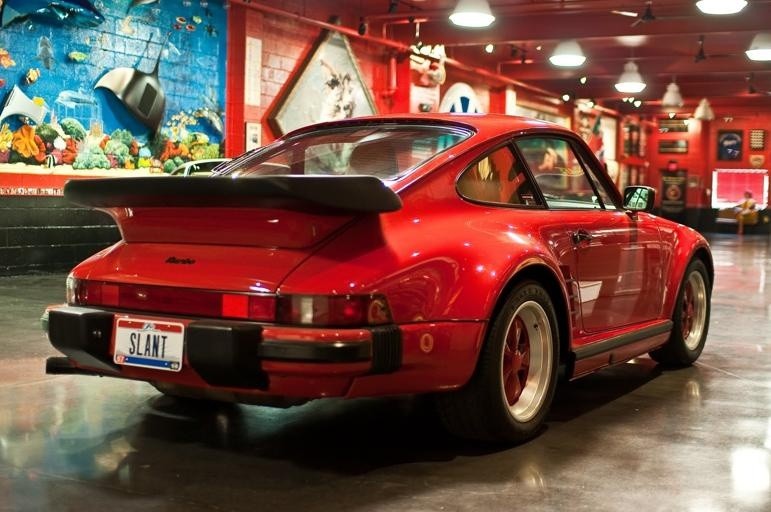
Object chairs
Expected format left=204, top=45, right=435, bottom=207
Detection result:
left=349, top=144, right=399, bottom=181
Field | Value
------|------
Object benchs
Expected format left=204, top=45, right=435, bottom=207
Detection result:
left=717, top=207, right=757, bottom=231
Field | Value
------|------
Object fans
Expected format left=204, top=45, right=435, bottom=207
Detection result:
left=609, top=1, right=695, bottom=28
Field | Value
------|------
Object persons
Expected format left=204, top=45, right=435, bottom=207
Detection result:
left=736, top=190, right=757, bottom=235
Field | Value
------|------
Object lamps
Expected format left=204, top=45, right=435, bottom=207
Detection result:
left=549, top=41, right=588, bottom=69
left=450, top=1, right=495, bottom=29
left=696, top=0, right=770, bottom=62
left=613, top=48, right=716, bottom=119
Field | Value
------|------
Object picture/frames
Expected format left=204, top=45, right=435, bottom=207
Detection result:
left=716, top=128, right=743, bottom=162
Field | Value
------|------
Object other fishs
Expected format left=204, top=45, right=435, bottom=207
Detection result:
left=46, top=154, right=58, bottom=169
left=167, top=85, right=224, bottom=147
left=55, top=91, right=100, bottom=109
left=92, top=30, right=176, bottom=147
left=33, top=96, right=44, bottom=106
left=168, top=42, right=181, bottom=56
left=195, top=55, right=219, bottom=72
left=121, top=0, right=162, bottom=35
left=167, top=0, right=220, bottom=40
left=0, top=34, right=92, bottom=86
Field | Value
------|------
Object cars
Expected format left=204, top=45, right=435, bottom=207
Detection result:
left=39, top=113, right=717, bottom=441
